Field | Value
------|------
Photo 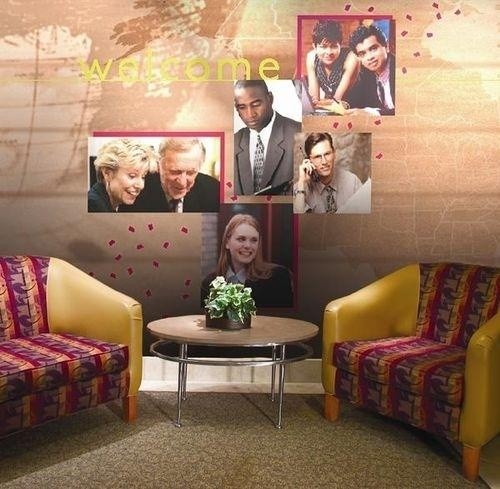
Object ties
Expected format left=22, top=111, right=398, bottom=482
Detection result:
left=326, top=188, right=337, bottom=215
left=377, top=81, right=386, bottom=105
left=169, top=198, right=180, bottom=211
left=254, top=135, right=264, bottom=193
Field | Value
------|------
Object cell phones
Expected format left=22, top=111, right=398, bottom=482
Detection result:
left=308, top=168, right=320, bottom=182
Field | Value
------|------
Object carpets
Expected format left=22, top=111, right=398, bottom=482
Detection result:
left=0, top=389, right=492, bottom=489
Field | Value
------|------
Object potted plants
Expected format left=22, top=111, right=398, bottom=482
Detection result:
left=202, top=275, right=258, bottom=329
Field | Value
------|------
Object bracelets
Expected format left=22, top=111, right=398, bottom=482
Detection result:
left=295, top=188, right=306, bottom=196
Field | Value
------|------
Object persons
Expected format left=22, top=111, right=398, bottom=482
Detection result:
left=295, top=132, right=365, bottom=215
left=305, top=18, right=359, bottom=113
left=203, top=214, right=293, bottom=311
left=233, top=79, right=301, bottom=196
left=344, top=25, right=394, bottom=116
left=138, top=139, right=219, bottom=213
left=87, top=139, right=154, bottom=213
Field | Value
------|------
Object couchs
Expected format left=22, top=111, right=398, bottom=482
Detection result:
left=0, top=253, right=145, bottom=444
left=317, top=261, right=500, bottom=482
